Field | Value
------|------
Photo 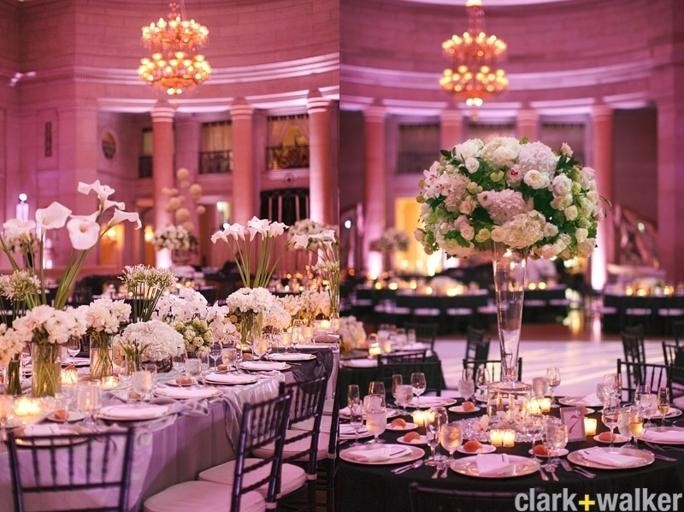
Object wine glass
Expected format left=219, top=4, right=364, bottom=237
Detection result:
left=346, top=367, right=671, bottom=464
left=67, top=335, right=80, bottom=367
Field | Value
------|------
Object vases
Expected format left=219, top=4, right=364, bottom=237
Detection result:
left=491, top=241, right=528, bottom=391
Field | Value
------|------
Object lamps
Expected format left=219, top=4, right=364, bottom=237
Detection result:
left=439, top=0, right=509, bottom=106
left=137, top=0, right=212, bottom=95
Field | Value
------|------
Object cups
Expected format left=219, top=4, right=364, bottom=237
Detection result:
left=117, top=315, right=414, bottom=402
left=0, top=379, right=105, bottom=425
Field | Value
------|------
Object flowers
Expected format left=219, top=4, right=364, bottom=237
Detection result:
left=152, top=223, right=191, bottom=251
left=211, top=216, right=308, bottom=291
left=413, top=133, right=612, bottom=261
left=0, top=179, right=143, bottom=396
left=365, top=224, right=409, bottom=253
left=287, top=217, right=340, bottom=320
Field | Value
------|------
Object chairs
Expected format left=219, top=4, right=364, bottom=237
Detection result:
left=0, top=265, right=684, bottom=512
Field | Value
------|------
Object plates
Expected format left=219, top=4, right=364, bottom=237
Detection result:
left=338, top=397, right=684, bottom=479
left=14, top=423, right=92, bottom=448
left=97, top=404, right=170, bottom=422
left=60, top=358, right=90, bottom=366
